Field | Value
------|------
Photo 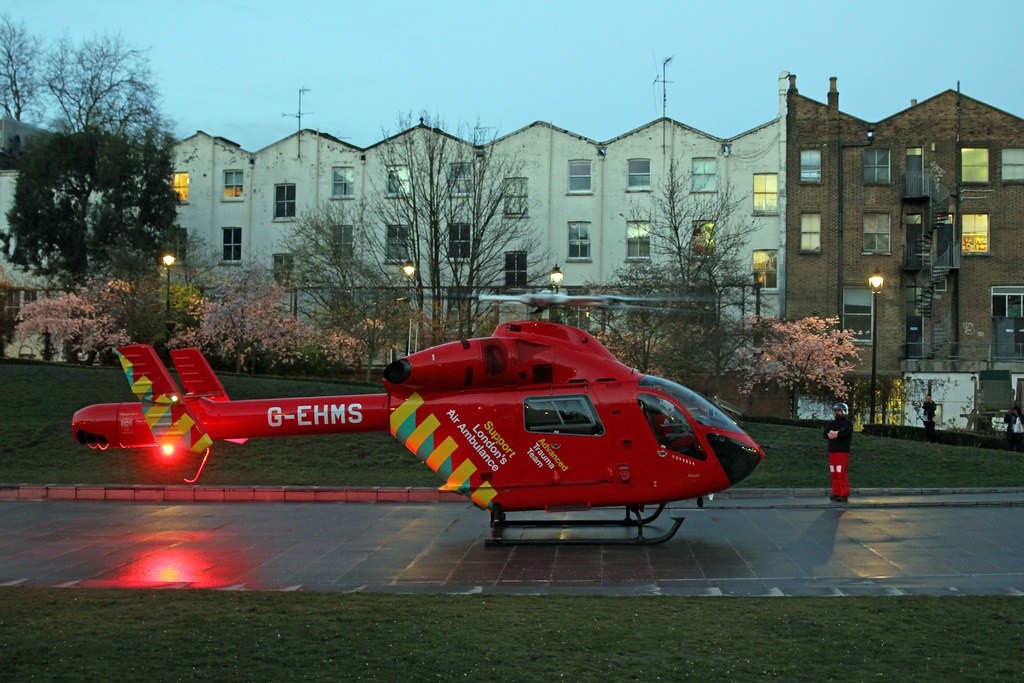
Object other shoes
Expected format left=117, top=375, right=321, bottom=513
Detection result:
left=829, top=495, right=840, bottom=500
left=836, top=496, right=848, bottom=501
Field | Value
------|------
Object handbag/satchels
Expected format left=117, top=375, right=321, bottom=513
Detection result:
left=1014, top=416, right=1024, bottom=433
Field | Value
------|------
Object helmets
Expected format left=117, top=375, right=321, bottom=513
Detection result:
left=832, top=402, right=850, bottom=417
left=658, top=398, right=677, bottom=413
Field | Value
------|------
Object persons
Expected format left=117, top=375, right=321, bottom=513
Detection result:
left=923, top=394, right=936, bottom=431
left=652, top=394, right=696, bottom=452
left=1004, top=405, right=1024, bottom=452
left=823, top=401, right=855, bottom=503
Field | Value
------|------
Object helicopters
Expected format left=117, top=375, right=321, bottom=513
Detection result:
left=68, top=293, right=768, bottom=550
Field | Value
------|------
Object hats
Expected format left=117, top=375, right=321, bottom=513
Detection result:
left=1011, top=405, right=1020, bottom=410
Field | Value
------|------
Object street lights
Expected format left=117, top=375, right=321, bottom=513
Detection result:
left=868, top=267, right=886, bottom=421
left=403, top=259, right=414, bottom=294
left=162, top=251, right=177, bottom=327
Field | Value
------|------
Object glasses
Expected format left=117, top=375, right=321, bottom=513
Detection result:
left=834, top=408, right=842, bottom=411
